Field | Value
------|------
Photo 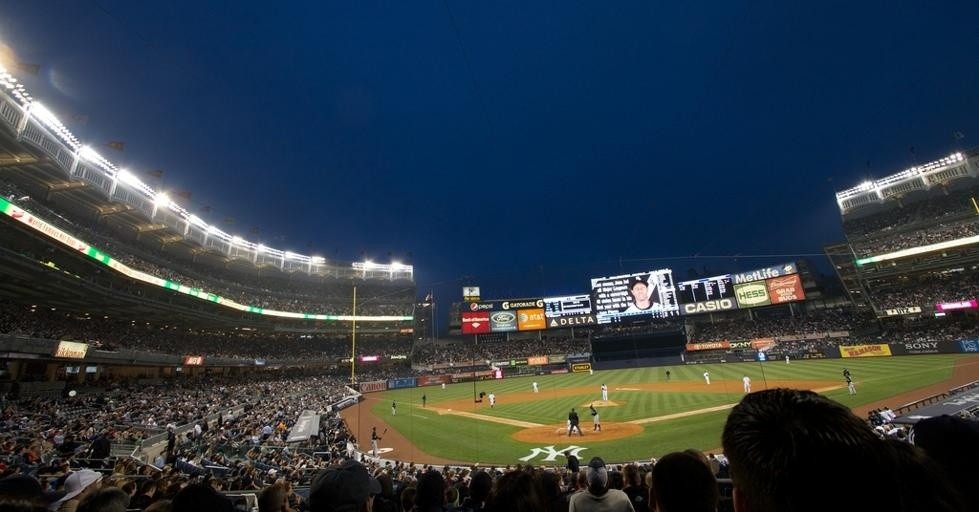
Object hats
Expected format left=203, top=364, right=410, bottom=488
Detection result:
left=586, top=457, right=608, bottom=488
left=56, top=469, right=103, bottom=502
left=311, top=459, right=382, bottom=501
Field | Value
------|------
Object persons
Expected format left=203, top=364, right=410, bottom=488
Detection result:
left=703, top=370, right=710, bottom=385
left=480, top=391, right=485, bottom=401
left=743, top=374, right=751, bottom=393
left=442, top=382, right=445, bottom=389
left=589, top=368, right=594, bottom=376
left=785, top=355, right=790, bottom=364
left=720, top=387, right=896, bottom=512
left=415, top=332, right=590, bottom=365
left=842, top=367, right=849, bottom=378
left=621, top=280, right=662, bottom=312
left=845, top=374, right=857, bottom=394
left=488, top=391, right=495, bottom=407
left=422, top=394, right=427, bottom=407
left=532, top=380, right=539, bottom=393
left=666, top=369, right=670, bottom=379
left=601, top=383, right=607, bottom=400
